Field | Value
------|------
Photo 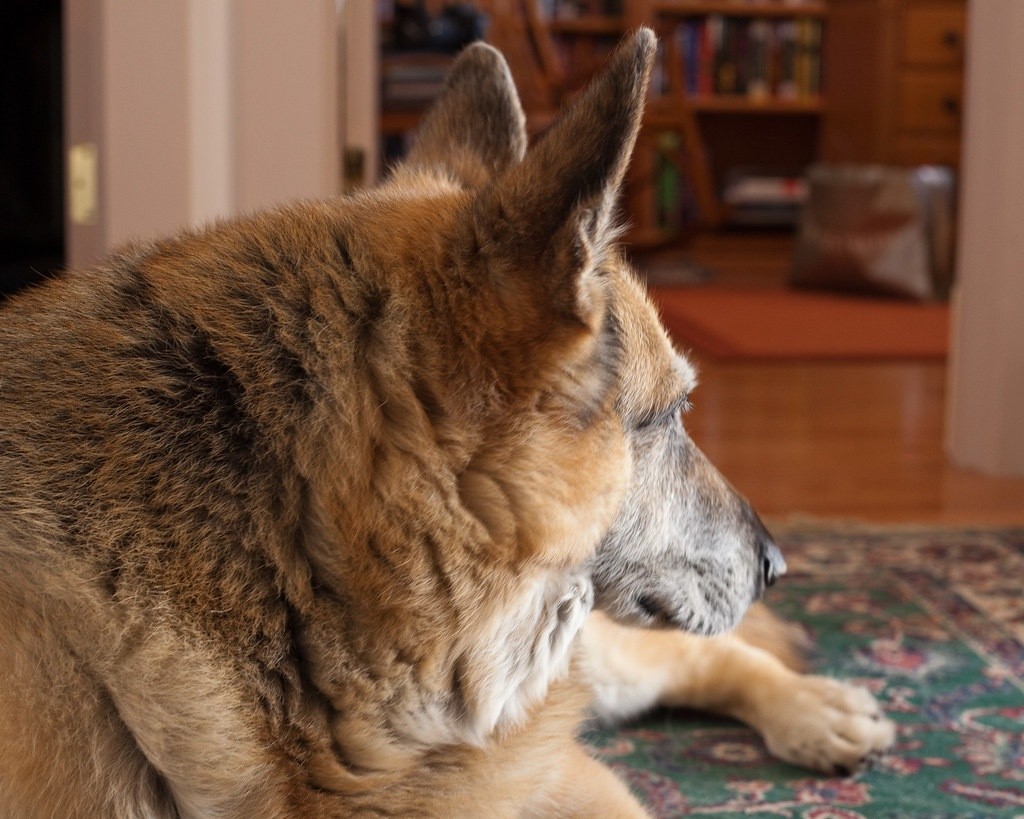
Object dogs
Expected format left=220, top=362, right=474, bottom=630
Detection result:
left=0, top=24, right=897, bottom=819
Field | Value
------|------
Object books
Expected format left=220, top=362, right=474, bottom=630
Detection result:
left=376, top=0, right=824, bottom=247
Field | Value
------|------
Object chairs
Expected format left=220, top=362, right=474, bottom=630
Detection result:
left=520, top=0, right=717, bottom=251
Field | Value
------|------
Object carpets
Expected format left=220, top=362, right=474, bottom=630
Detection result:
left=580, top=509, right=1024, bottom=818
left=648, top=280, right=952, bottom=367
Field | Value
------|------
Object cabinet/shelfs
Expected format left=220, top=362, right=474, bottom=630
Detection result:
left=601, top=0, right=969, bottom=233
